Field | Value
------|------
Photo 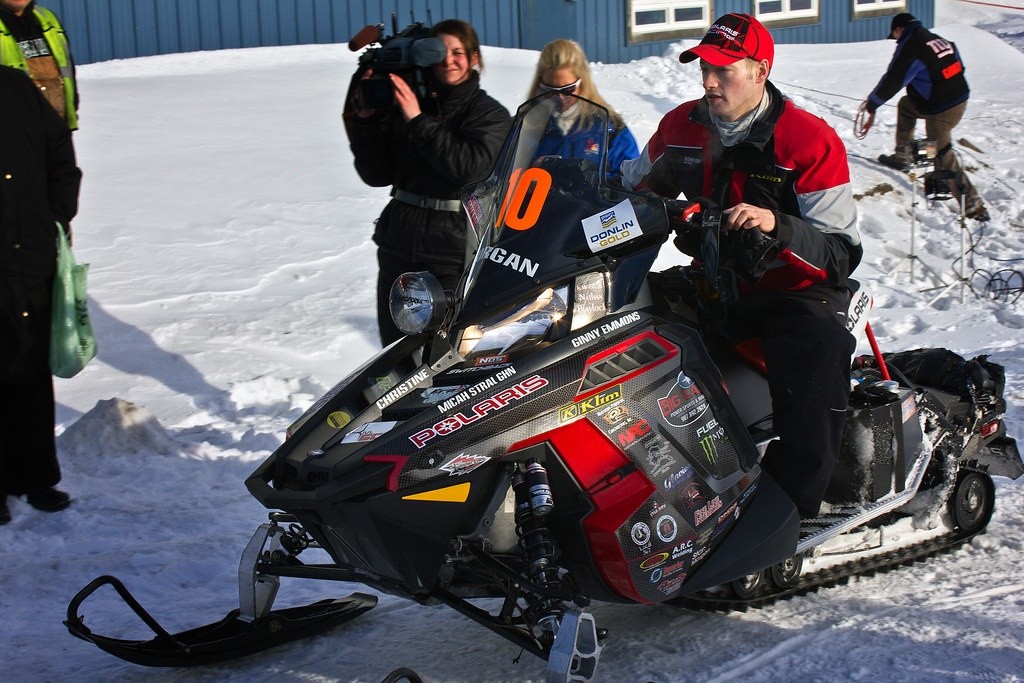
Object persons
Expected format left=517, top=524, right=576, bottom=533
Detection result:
left=0, top=63, right=83, bottom=526
left=341, top=19, right=513, bottom=349
left=494, top=39, right=640, bottom=191
left=532, top=13, right=863, bottom=519
left=854, top=12, right=992, bottom=222
left=0, top=0, right=80, bottom=134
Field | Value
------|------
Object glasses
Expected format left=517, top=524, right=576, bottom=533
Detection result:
left=538, top=79, right=582, bottom=99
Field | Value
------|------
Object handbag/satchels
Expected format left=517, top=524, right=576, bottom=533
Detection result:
left=49, top=221, right=97, bottom=378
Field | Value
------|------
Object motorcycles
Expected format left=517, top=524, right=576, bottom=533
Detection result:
left=64, top=88, right=1024, bottom=683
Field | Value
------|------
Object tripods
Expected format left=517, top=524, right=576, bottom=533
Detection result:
left=888, top=163, right=983, bottom=309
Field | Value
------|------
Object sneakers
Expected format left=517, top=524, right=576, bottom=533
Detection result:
left=967, top=205, right=990, bottom=222
left=879, top=154, right=914, bottom=172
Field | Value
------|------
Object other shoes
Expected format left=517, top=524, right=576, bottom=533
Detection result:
left=7, top=480, right=73, bottom=512
left=0, top=492, right=11, bottom=525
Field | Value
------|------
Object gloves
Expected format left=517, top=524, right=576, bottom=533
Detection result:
left=866, top=99, right=879, bottom=113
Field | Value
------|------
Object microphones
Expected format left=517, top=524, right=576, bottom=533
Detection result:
left=349, top=23, right=385, bottom=52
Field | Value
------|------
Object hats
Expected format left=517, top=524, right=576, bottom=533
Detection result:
left=679, top=13, right=774, bottom=68
left=888, top=11, right=914, bottom=39
